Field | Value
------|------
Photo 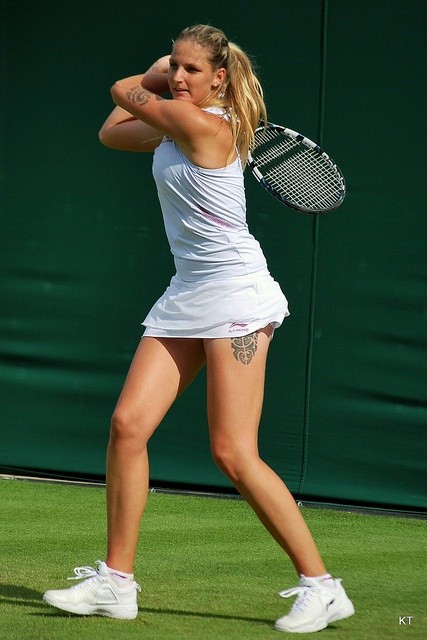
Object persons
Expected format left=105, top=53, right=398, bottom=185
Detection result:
left=41, top=23, right=356, bottom=634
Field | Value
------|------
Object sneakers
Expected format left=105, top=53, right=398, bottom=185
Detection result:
left=42, top=560, right=141, bottom=620
left=275, top=574, right=355, bottom=633
